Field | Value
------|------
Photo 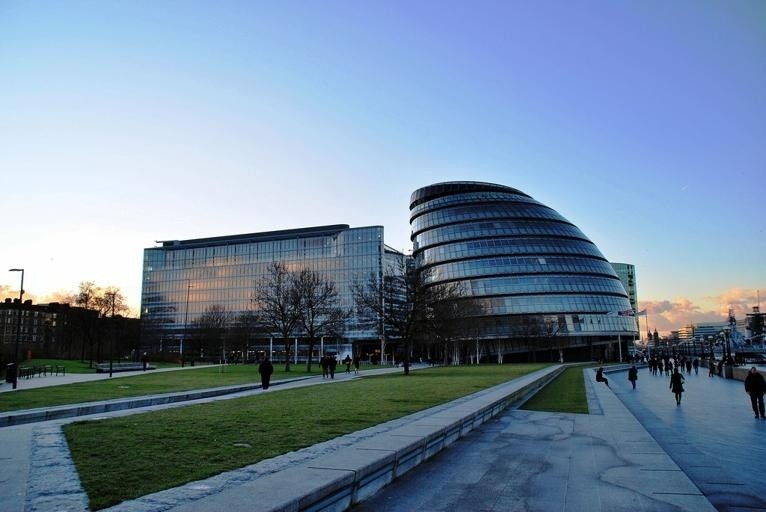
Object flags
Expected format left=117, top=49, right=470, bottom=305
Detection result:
left=637, top=309, right=647, bottom=315
left=607, top=310, right=618, bottom=317
left=622, top=309, right=634, bottom=317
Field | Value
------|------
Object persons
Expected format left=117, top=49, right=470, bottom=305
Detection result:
left=318, top=354, right=360, bottom=379
left=259, top=357, right=273, bottom=390
left=181, top=354, right=195, bottom=368
left=596, top=354, right=766, bottom=419
left=141, top=353, right=149, bottom=372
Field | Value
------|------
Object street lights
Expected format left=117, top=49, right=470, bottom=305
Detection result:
left=719, top=326, right=731, bottom=359
left=9, top=268, right=24, bottom=389
left=678, top=336, right=716, bottom=361
